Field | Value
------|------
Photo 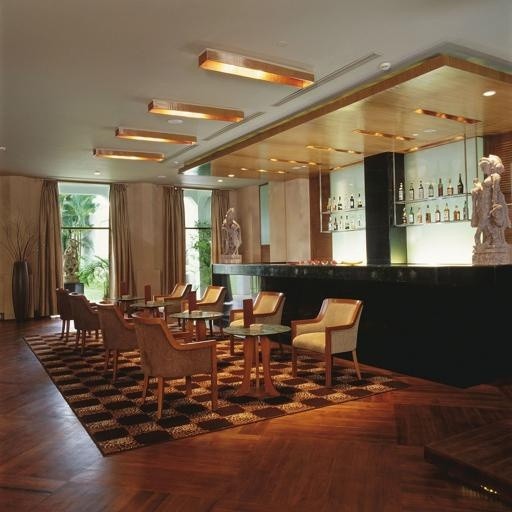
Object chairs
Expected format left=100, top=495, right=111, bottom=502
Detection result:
left=290, top=298, right=362, bottom=387
left=230, top=291, right=288, bottom=355
left=56, top=284, right=227, bottom=384
left=134, top=321, right=217, bottom=419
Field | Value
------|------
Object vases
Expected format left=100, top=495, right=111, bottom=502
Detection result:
left=12, top=260, right=32, bottom=325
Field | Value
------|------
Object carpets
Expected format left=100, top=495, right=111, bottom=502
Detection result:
left=21, top=323, right=413, bottom=456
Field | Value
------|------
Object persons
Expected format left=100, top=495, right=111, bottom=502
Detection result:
left=473, top=154, right=512, bottom=246
left=222, top=208, right=241, bottom=256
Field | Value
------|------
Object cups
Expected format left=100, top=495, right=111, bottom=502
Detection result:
left=296, top=255, right=336, bottom=264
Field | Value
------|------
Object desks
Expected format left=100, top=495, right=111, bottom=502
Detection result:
left=222, top=324, right=292, bottom=399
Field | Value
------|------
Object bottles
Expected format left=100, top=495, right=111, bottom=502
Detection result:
left=328, top=215, right=362, bottom=231
left=326, top=193, right=363, bottom=211
left=402, top=201, right=469, bottom=224
left=399, top=172, right=464, bottom=200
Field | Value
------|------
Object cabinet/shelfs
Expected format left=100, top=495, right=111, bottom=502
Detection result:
left=319, top=167, right=365, bottom=233
left=393, top=135, right=480, bottom=227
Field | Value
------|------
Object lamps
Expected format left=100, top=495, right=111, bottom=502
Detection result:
left=93, top=47, right=315, bottom=162
